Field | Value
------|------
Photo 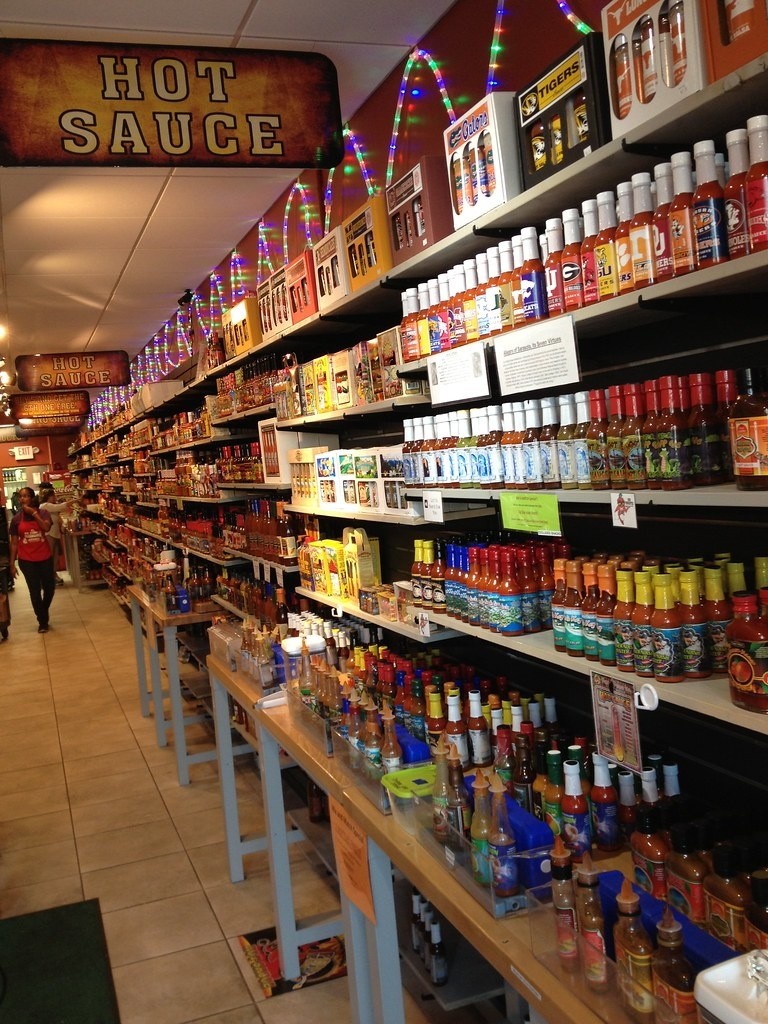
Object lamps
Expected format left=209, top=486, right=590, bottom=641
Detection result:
left=0, top=355, right=13, bottom=416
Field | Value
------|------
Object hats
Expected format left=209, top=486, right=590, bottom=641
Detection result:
left=39, top=482, right=55, bottom=489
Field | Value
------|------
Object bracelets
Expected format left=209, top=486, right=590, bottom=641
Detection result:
left=32, top=510, right=37, bottom=516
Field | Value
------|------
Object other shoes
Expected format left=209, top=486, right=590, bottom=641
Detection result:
left=38, top=623, right=49, bottom=632
left=54, top=577, right=64, bottom=583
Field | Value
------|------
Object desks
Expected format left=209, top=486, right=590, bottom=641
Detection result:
left=206, top=653, right=377, bottom=1024
left=342, top=785, right=768, bottom=1024
left=127, top=584, right=229, bottom=786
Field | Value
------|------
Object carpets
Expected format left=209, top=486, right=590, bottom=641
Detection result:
left=0, top=896, right=121, bottom=1024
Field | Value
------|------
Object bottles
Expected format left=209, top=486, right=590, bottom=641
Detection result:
left=75, top=113, right=768, bottom=1024
left=2, top=471, right=15, bottom=481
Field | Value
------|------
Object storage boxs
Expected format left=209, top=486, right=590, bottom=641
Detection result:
left=693, top=949, right=768, bottom=1024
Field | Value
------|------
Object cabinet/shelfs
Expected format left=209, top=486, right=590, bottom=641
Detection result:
left=65, top=326, right=273, bottom=747
left=272, top=50, right=768, bottom=736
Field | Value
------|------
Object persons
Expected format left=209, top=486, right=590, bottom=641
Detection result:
left=35, top=482, right=82, bottom=586
left=8, top=487, right=56, bottom=633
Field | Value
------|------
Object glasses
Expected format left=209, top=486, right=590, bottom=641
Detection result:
left=18, top=494, right=31, bottom=498
